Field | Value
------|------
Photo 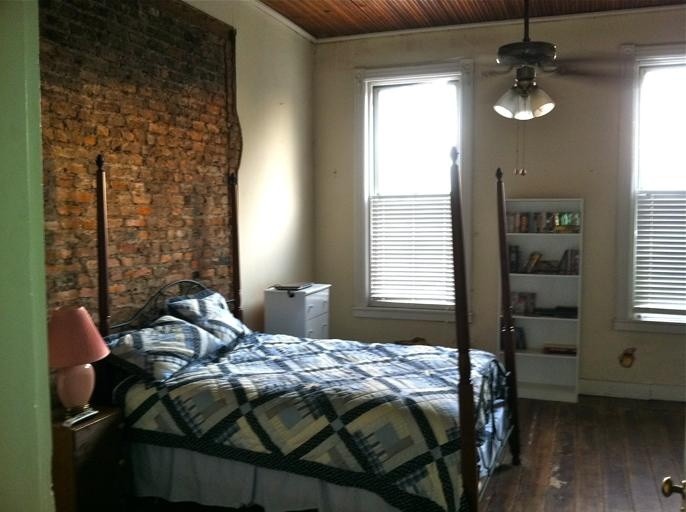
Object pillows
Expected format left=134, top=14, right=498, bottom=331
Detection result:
left=168, top=289, right=253, bottom=346
left=102, top=313, right=229, bottom=387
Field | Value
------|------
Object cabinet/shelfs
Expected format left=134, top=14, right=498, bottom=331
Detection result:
left=498, top=199, right=584, bottom=402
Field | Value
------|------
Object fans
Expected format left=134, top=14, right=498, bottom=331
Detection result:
left=451, top=0, right=642, bottom=87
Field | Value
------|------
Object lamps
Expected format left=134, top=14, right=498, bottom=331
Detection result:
left=493, top=68, right=555, bottom=175
left=49, top=306, right=111, bottom=412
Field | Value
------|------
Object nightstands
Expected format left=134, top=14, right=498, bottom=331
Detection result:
left=264, top=283, right=331, bottom=337
left=51, top=407, right=123, bottom=512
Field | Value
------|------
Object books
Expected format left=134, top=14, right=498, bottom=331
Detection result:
left=506, top=210, right=579, bottom=355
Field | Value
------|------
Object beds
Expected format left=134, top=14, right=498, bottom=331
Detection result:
left=96, top=148, right=521, bottom=511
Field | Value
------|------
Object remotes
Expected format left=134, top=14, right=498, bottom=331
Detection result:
left=62, top=409, right=99, bottom=427
